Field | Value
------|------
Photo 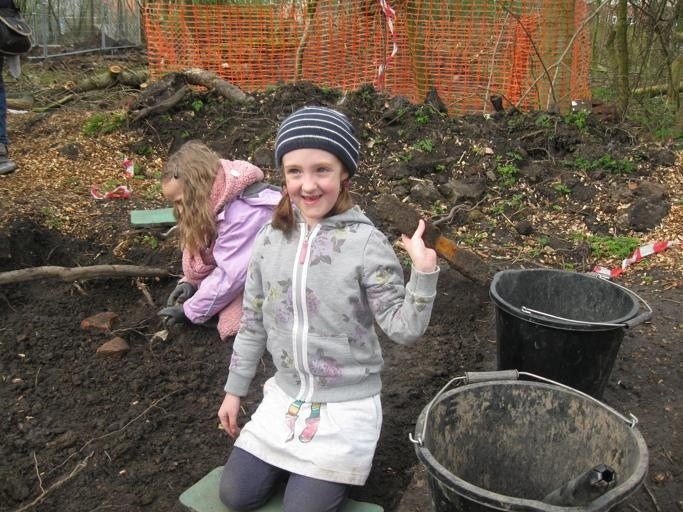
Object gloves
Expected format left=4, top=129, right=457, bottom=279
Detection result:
left=158, top=305, right=192, bottom=334
left=167, top=282, right=192, bottom=306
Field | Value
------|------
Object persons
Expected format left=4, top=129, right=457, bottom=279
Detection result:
left=0, top=1, right=21, bottom=178
left=215, top=104, right=440, bottom=512
left=155, top=140, right=297, bottom=341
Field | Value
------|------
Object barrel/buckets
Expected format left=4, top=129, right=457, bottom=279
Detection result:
left=488, top=267, right=654, bottom=402
left=409, top=368, right=651, bottom=511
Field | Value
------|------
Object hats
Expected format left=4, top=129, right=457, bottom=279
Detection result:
left=275, top=105, right=361, bottom=178
